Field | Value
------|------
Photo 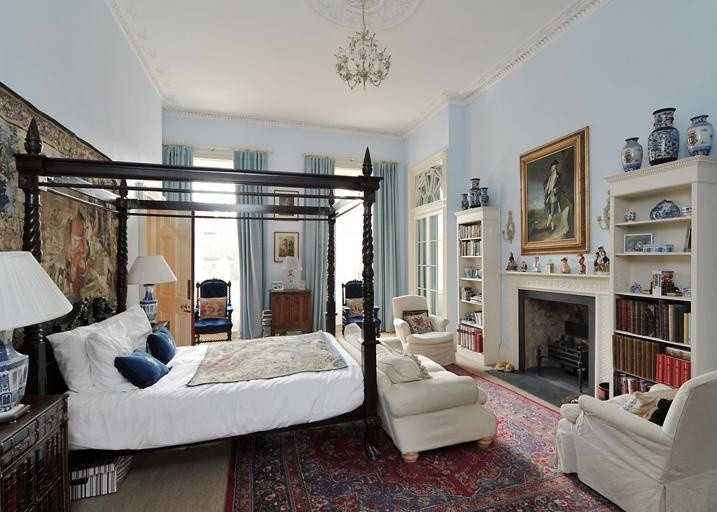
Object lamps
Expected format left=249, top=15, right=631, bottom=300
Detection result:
left=125, top=255, right=178, bottom=327
left=333, top=0, right=393, bottom=90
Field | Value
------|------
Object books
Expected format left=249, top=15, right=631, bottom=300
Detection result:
left=459, top=224, right=482, bottom=353
left=613, top=297, right=692, bottom=401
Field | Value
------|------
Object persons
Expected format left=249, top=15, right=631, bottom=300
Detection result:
left=282, top=238, right=294, bottom=251
left=542, top=160, right=566, bottom=233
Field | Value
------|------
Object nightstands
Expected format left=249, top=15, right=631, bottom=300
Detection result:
left=152, top=319, right=170, bottom=333
left=0, top=393, right=70, bottom=511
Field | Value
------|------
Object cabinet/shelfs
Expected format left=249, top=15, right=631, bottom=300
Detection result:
left=267, top=287, right=313, bottom=336
left=602, top=155, right=716, bottom=398
left=452, top=206, right=501, bottom=367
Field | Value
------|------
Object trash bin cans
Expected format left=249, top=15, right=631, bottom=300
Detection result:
left=598, top=383, right=610, bottom=401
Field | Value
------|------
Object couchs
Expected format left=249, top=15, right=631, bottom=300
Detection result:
left=338, top=336, right=497, bottom=463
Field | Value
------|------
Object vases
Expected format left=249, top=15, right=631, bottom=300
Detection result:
left=459, top=177, right=489, bottom=211
left=620, top=107, right=712, bottom=173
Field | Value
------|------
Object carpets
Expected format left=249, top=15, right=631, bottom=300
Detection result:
left=224, top=362, right=627, bottom=512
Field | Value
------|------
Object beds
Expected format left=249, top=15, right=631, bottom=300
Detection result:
left=14, top=116, right=383, bottom=460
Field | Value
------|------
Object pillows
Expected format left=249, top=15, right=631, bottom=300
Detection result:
left=621, top=388, right=677, bottom=426
left=346, top=298, right=364, bottom=318
left=45, top=306, right=177, bottom=394
left=394, top=349, right=431, bottom=379
left=198, top=296, right=226, bottom=320
left=403, top=309, right=433, bottom=335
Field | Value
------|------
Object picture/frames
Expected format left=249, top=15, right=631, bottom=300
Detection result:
left=274, top=189, right=300, bottom=221
left=519, top=125, right=591, bottom=255
left=623, top=233, right=653, bottom=252
left=273, top=231, right=299, bottom=262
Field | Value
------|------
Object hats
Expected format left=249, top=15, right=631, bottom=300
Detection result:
left=550, top=159, right=559, bottom=168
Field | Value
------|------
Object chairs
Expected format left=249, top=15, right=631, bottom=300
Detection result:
left=392, top=294, right=457, bottom=368
left=340, top=280, right=381, bottom=337
left=556, top=370, right=717, bottom=512
left=193, top=279, right=233, bottom=344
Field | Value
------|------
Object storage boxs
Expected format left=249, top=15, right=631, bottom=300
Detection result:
left=69, top=453, right=135, bottom=501
